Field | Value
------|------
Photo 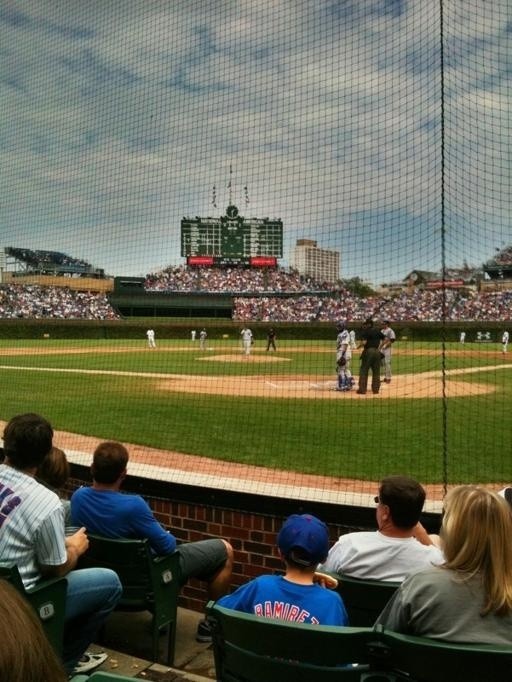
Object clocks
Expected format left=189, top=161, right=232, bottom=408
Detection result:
left=224, top=205, right=239, bottom=219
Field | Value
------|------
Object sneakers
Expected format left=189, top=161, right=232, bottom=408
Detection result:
left=72, top=650, right=108, bottom=675
left=196, top=616, right=211, bottom=643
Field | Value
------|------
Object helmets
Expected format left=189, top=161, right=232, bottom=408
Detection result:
left=336, top=319, right=345, bottom=332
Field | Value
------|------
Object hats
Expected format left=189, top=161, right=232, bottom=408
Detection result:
left=275, top=514, right=329, bottom=567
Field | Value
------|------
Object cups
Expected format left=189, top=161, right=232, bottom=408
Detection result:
left=63, top=525, right=80, bottom=538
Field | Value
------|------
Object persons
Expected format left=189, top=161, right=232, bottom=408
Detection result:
left=0, top=265, right=118, bottom=320
left=215, top=513, right=350, bottom=668
left=373, top=486, right=512, bottom=644
left=68, top=442, right=235, bottom=642
left=0, top=413, right=123, bottom=674
left=144, top=266, right=343, bottom=292
left=0, top=579, right=68, bottom=682
left=350, top=325, right=395, bottom=351
left=34, top=445, right=71, bottom=523
left=461, top=328, right=509, bottom=352
left=146, top=327, right=155, bottom=348
left=191, top=327, right=276, bottom=354
left=233, top=289, right=511, bottom=321
left=336, top=320, right=355, bottom=391
left=379, top=321, right=396, bottom=384
left=317, top=475, right=447, bottom=581
left=356, top=319, right=389, bottom=394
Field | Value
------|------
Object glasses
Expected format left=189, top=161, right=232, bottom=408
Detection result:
left=374, top=496, right=386, bottom=504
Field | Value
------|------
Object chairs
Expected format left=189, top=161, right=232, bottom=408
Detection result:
left=0, top=498, right=510, bottom=680
left=4, top=244, right=102, bottom=281
